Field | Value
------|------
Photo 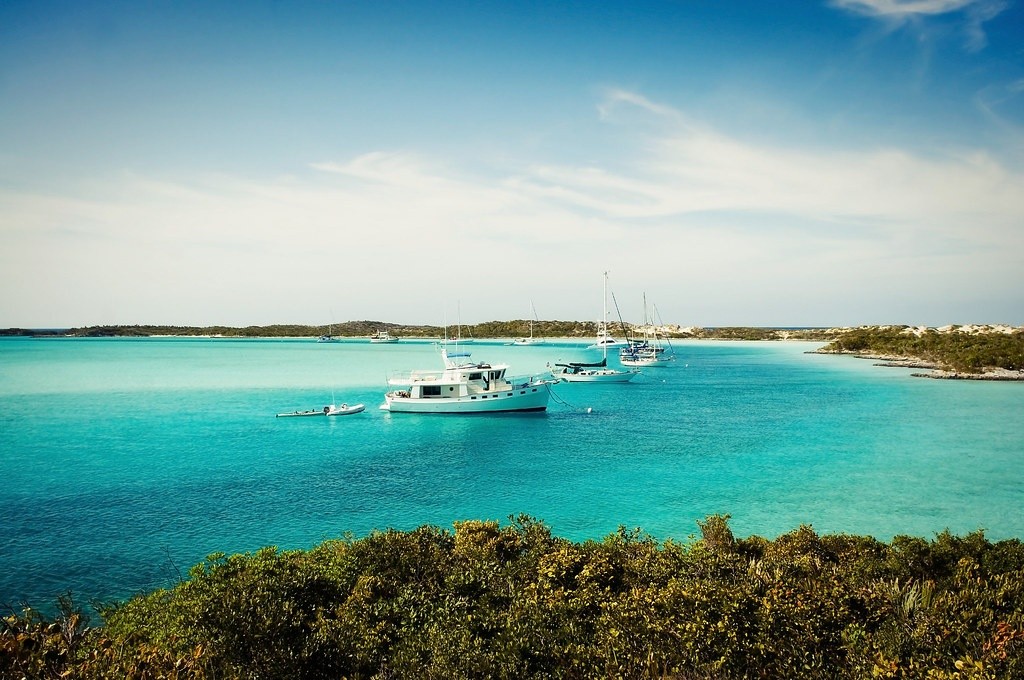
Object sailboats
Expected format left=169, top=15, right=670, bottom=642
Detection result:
left=595, top=288, right=676, bottom=367
left=547, top=272, right=644, bottom=383
left=441, top=298, right=473, bottom=345
left=513, top=298, right=545, bottom=346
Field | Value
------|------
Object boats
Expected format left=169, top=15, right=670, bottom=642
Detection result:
left=316, top=334, right=341, bottom=343
left=377, top=340, right=562, bottom=414
left=370, top=329, right=399, bottom=344
